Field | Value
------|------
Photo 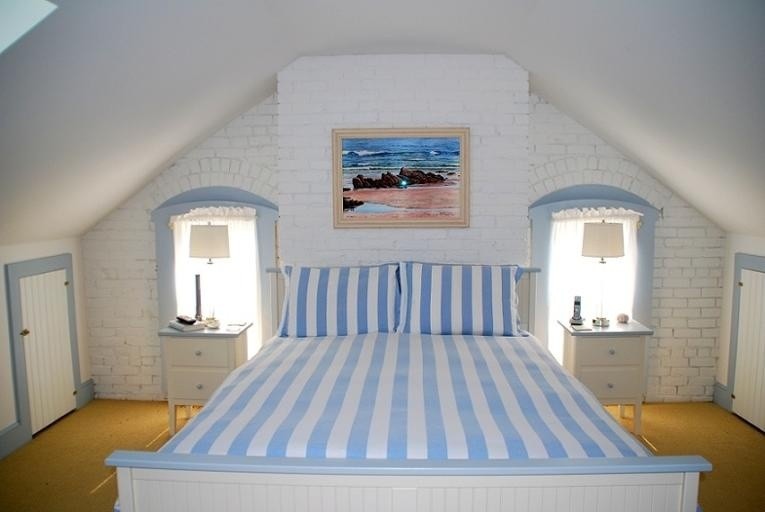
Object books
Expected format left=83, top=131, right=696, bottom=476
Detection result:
left=169, top=320, right=205, bottom=331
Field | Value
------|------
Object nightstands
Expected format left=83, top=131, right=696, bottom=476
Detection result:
left=558, top=318, right=653, bottom=436
left=158, top=321, right=253, bottom=436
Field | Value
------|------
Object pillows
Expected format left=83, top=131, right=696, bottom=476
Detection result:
left=401, top=261, right=518, bottom=336
left=280, top=262, right=400, bottom=336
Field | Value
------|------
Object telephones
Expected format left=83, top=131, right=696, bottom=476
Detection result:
left=570, top=295, right=584, bottom=325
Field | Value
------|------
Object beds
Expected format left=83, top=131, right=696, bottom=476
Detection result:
left=104, top=259, right=712, bottom=512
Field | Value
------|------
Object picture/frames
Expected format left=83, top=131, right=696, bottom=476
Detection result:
left=331, top=127, right=469, bottom=229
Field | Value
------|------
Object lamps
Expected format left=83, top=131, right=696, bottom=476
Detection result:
left=581, top=217, right=625, bottom=264
left=190, top=216, right=230, bottom=264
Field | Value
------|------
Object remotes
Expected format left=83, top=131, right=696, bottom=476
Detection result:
left=176, top=315, right=196, bottom=324
